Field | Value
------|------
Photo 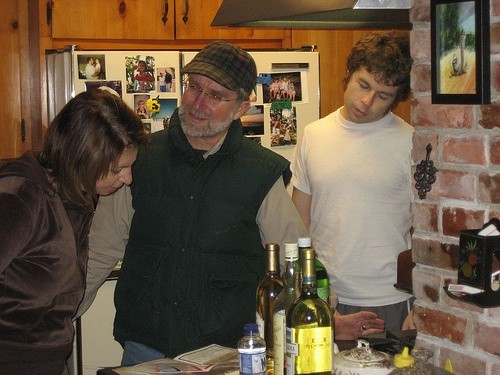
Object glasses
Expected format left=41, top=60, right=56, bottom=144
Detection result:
left=181, top=76, right=239, bottom=105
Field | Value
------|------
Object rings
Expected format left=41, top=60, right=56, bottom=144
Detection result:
left=362, top=325, right=367, bottom=330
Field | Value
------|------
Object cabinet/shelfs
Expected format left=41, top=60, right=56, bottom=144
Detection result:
left=28, top=0, right=291, bottom=51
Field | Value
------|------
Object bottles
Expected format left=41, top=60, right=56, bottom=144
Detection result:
left=237, top=237, right=335, bottom=375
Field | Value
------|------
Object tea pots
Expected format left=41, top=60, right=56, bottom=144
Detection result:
left=334, top=339, right=394, bottom=375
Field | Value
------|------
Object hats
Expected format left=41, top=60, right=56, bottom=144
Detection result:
left=181, top=40, right=257, bottom=96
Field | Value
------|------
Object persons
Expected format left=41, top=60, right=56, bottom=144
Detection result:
left=159, top=69, right=172, bottom=92
left=290, top=31, right=413, bottom=339
left=74, top=41, right=385, bottom=365
left=135, top=100, right=148, bottom=119
left=269, top=78, right=295, bottom=102
left=0, top=88, right=150, bottom=375
left=85, top=57, right=100, bottom=78
left=270, top=117, right=291, bottom=144
left=131, top=60, right=150, bottom=90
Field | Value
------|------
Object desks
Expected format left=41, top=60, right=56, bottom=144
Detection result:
left=96, top=340, right=455, bottom=375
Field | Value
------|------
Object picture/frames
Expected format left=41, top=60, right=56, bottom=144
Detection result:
left=429, top=0, right=491, bottom=105
